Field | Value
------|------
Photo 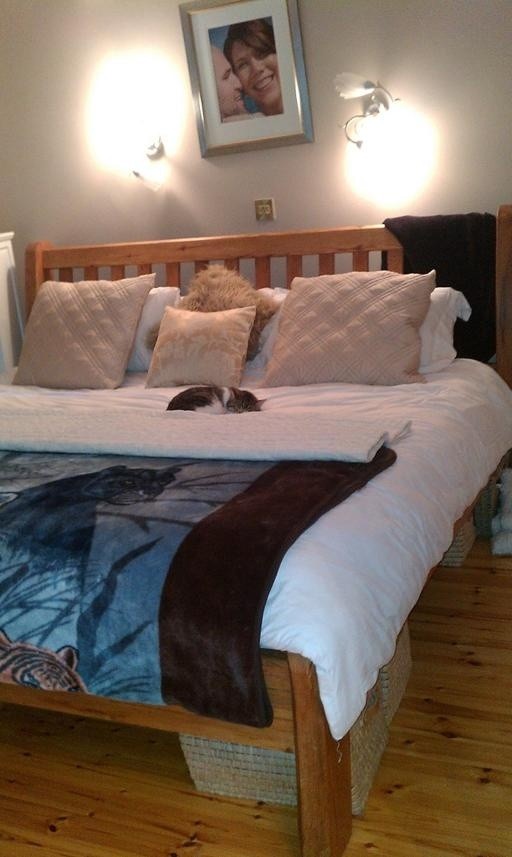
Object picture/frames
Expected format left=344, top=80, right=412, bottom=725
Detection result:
left=177, top=0, right=314, bottom=156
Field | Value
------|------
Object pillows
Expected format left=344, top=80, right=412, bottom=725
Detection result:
left=142, top=302, right=260, bottom=389
left=145, top=261, right=281, bottom=363
left=246, top=283, right=470, bottom=381
left=257, top=267, right=436, bottom=386
left=127, top=285, right=181, bottom=371
left=11, top=270, right=156, bottom=386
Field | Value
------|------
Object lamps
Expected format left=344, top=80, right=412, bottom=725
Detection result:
left=331, top=70, right=441, bottom=174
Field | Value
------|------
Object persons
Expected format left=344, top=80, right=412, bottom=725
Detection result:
left=209, top=43, right=266, bottom=124
left=221, top=18, right=286, bottom=116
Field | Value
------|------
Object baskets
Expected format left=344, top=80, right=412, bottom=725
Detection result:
left=379, top=618, right=413, bottom=725
left=178, top=674, right=389, bottom=815
left=439, top=513, right=476, bottom=567
left=474, top=454, right=510, bottom=538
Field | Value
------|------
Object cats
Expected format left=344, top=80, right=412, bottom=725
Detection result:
left=164, top=384, right=267, bottom=415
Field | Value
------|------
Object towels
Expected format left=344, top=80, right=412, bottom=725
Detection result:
left=381, top=211, right=498, bottom=362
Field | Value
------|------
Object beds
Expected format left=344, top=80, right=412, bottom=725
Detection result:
left=0, top=200, right=512, bottom=852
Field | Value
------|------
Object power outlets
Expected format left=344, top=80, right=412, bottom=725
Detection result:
left=255, top=199, right=275, bottom=222
